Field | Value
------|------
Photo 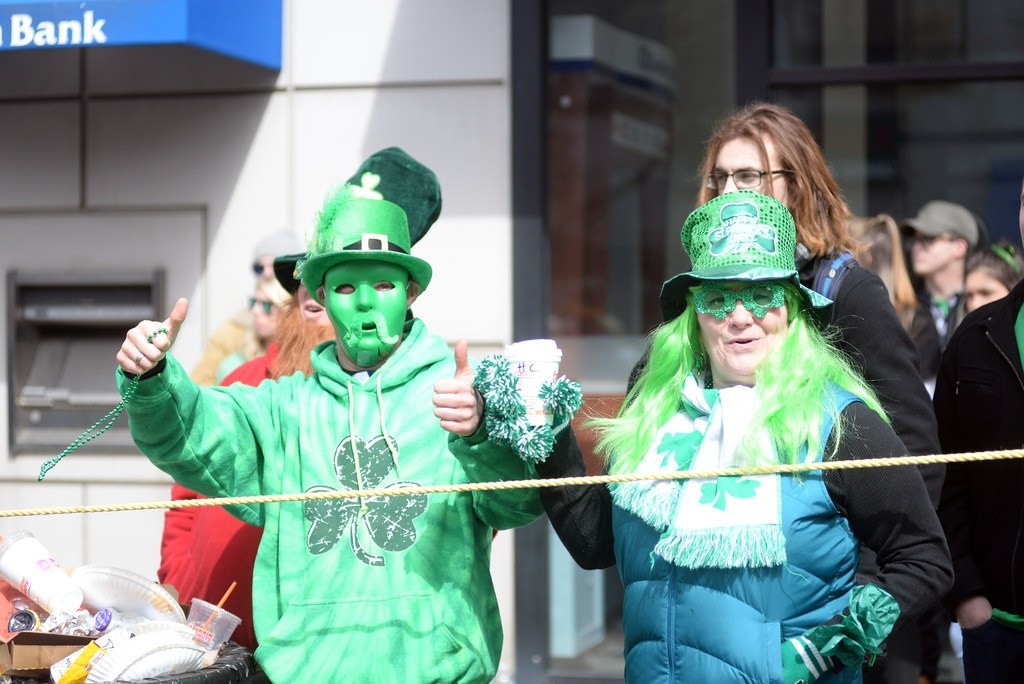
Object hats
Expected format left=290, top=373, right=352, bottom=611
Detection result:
left=659, top=189, right=837, bottom=332
left=298, top=193, right=432, bottom=304
left=901, top=200, right=978, bottom=252
left=272, top=147, right=443, bottom=300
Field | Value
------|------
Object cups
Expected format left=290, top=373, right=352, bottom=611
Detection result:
left=506, top=337, right=563, bottom=429
left=185, top=597, right=242, bottom=668
left=0, top=530, right=83, bottom=614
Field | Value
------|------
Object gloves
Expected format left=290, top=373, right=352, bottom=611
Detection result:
left=779, top=631, right=835, bottom=684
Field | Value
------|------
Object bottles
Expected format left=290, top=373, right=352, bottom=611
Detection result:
left=49, top=618, right=151, bottom=684
left=95, top=610, right=151, bottom=632
left=9, top=611, right=42, bottom=631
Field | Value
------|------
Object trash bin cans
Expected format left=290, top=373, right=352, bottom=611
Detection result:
left=0, top=551, right=257, bottom=684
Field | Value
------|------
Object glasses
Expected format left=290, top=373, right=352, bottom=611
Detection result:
left=707, top=165, right=797, bottom=192
left=694, top=282, right=786, bottom=320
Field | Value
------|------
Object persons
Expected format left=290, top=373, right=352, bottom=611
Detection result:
left=475, top=191, right=954, bottom=683
left=155, top=147, right=444, bottom=648
left=115, top=190, right=543, bottom=684
left=627, top=103, right=1023, bottom=684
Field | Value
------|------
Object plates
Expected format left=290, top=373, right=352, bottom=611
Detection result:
left=73, top=565, right=208, bottom=683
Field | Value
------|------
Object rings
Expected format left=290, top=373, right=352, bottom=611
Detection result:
left=135, top=353, right=144, bottom=365
